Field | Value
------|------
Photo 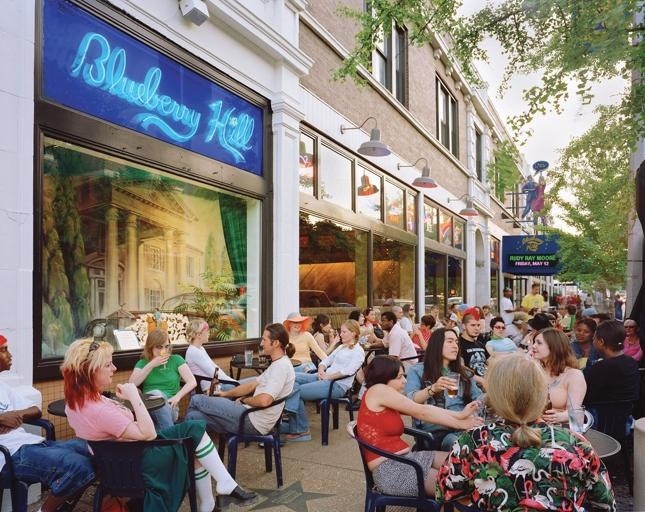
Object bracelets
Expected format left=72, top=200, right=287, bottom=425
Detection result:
left=239, top=397, right=246, bottom=404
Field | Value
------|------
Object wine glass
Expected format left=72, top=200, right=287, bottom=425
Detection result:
left=158, top=348, right=170, bottom=371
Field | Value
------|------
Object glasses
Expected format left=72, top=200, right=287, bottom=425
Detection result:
left=85, top=342, right=100, bottom=358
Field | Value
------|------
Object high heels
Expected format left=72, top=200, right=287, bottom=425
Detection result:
left=216, top=485, right=259, bottom=510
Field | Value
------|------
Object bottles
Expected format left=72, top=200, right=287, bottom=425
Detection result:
left=544, top=393, right=554, bottom=416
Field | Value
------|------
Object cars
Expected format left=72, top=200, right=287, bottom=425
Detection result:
left=235, top=289, right=357, bottom=322
left=371, top=294, right=463, bottom=310
left=85, top=291, right=242, bottom=351
left=490, top=298, right=497, bottom=308
left=614, top=290, right=627, bottom=303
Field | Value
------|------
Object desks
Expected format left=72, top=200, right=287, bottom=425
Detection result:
left=482, top=417, right=622, bottom=459
left=230, top=357, right=302, bottom=381
left=47, top=392, right=165, bottom=421
left=363, top=347, right=384, bottom=367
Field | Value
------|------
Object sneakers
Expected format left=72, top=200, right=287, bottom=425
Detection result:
left=287, top=431, right=311, bottom=442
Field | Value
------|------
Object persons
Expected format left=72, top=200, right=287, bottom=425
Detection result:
left=521, top=175, right=536, bottom=219
left=60, top=337, right=259, bottom=512
left=280, top=284, right=643, bottom=510
left=128, top=327, right=198, bottom=432
left=184, top=323, right=295, bottom=436
left=532, top=177, right=546, bottom=225
left=0, top=335, right=97, bottom=512
left=184, top=320, right=257, bottom=396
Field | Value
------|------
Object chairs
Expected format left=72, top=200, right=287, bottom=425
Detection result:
left=217, top=387, right=290, bottom=489
left=399, top=354, right=425, bottom=375
left=346, top=419, right=453, bottom=512
left=319, top=367, right=358, bottom=447
left=193, top=373, right=240, bottom=397
left=84, top=437, right=198, bottom=512
left=0, top=416, right=56, bottom=512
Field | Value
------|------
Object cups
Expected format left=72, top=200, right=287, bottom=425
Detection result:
left=244, top=348, right=253, bottom=366
left=258, top=345, right=266, bottom=362
left=446, top=370, right=460, bottom=399
left=567, top=405, right=587, bottom=435
left=333, top=328, right=337, bottom=335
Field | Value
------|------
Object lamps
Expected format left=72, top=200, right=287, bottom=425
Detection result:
left=448, top=193, right=477, bottom=218
left=359, top=169, right=379, bottom=196
left=396, top=158, right=437, bottom=190
left=340, top=116, right=392, bottom=158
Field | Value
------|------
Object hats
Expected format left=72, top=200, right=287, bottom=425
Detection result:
left=283, top=311, right=312, bottom=332
left=528, top=313, right=550, bottom=331
